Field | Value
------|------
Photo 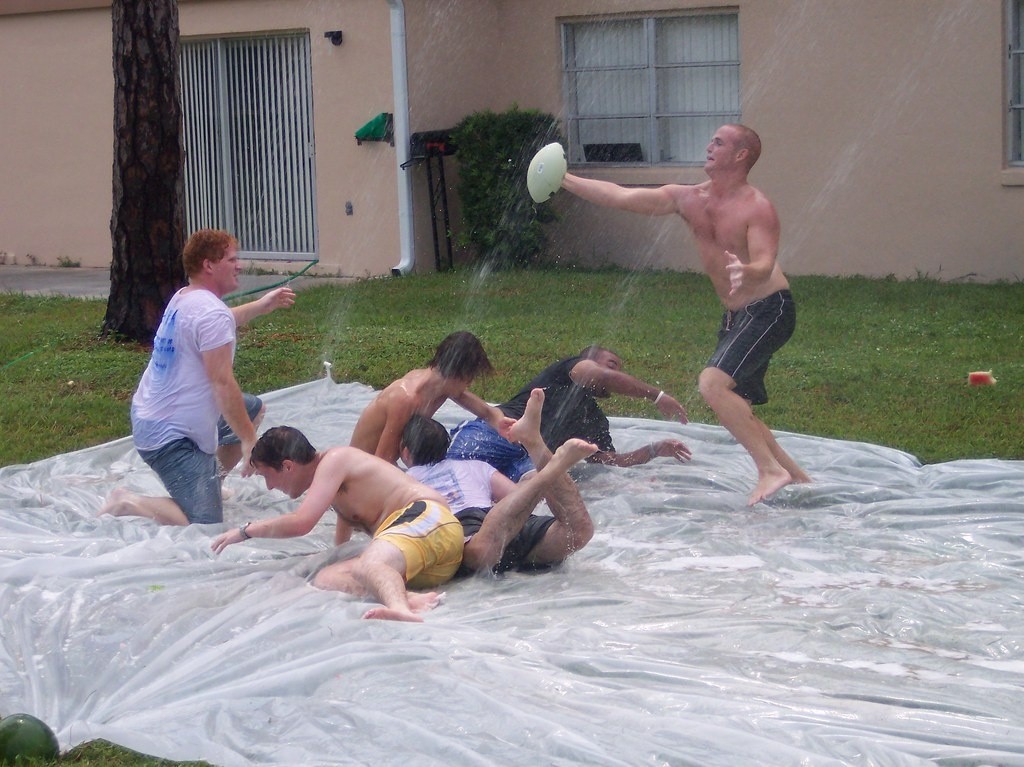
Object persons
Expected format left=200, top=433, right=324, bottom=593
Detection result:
left=562, top=123, right=811, bottom=506
left=349, top=330, right=517, bottom=474
left=98, top=229, right=296, bottom=526
left=446, top=346, right=692, bottom=486
left=211, top=426, right=465, bottom=622
left=398, top=389, right=598, bottom=574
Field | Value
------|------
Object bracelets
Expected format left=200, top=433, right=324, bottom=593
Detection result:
left=240, top=522, right=250, bottom=539
left=654, top=391, right=664, bottom=405
left=649, top=442, right=657, bottom=459
